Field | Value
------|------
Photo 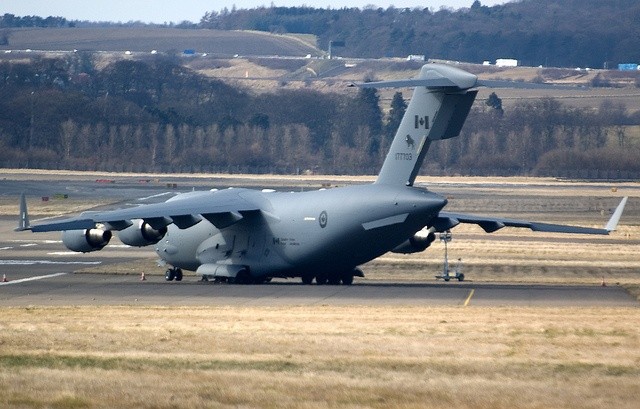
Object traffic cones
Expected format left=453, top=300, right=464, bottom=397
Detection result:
left=2, top=274, right=7, bottom=281
left=601, top=276, right=605, bottom=286
left=141, top=271, right=146, bottom=281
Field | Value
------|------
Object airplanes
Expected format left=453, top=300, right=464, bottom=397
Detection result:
left=13, top=62, right=629, bottom=285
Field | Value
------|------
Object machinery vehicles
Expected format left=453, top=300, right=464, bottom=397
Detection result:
left=435, top=231, right=465, bottom=282
